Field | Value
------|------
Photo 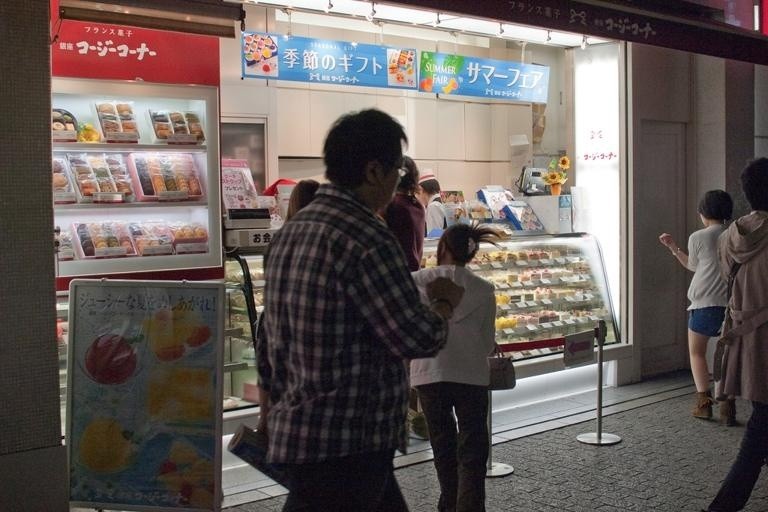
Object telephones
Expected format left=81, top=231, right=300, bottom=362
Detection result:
left=518, top=167, right=547, bottom=194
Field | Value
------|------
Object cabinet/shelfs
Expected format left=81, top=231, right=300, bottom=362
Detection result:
left=230, top=232, right=620, bottom=403
left=52, top=77, right=222, bottom=276
left=56, top=291, right=260, bottom=437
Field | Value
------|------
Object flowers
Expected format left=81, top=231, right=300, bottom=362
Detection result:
left=542, top=156, right=569, bottom=186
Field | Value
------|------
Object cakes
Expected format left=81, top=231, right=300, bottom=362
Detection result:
left=495, top=248, right=609, bottom=341
left=465, top=252, right=495, bottom=284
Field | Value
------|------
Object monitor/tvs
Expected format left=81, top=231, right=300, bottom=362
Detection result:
left=520, top=167, right=549, bottom=197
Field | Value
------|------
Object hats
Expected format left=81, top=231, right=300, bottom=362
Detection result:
left=417, top=168, right=436, bottom=185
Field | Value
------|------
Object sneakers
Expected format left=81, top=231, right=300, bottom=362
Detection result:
left=456, top=492, right=485, bottom=511
left=437, top=493, right=458, bottom=512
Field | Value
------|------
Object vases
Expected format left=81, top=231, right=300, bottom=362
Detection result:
left=550, top=183, right=562, bottom=196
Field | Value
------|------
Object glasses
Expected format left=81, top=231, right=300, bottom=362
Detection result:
left=376, top=159, right=410, bottom=176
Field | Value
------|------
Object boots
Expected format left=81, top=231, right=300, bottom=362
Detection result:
left=692, top=390, right=713, bottom=419
left=720, top=399, right=737, bottom=427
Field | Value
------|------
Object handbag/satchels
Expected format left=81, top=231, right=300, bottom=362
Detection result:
left=405, top=407, right=430, bottom=442
left=487, top=345, right=516, bottom=391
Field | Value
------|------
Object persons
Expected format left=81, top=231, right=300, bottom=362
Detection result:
left=281, top=155, right=450, bottom=272
left=251, top=109, right=465, bottom=511
left=380, top=157, right=434, bottom=442
left=707, top=156, right=768, bottom=512
left=409, top=219, right=496, bottom=511
left=658, top=189, right=737, bottom=428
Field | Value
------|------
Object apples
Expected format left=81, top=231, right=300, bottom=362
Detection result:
left=83, top=333, right=137, bottom=384
left=168, top=225, right=207, bottom=240
left=129, top=223, right=171, bottom=253
left=79, top=418, right=133, bottom=475
left=74, top=222, right=135, bottom=256
left=133, top=154, right=201, bottom=195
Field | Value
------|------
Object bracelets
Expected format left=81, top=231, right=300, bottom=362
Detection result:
left=429, top=297, right=454, bottom=321
left=672, top=248, right=681, bottom=256
